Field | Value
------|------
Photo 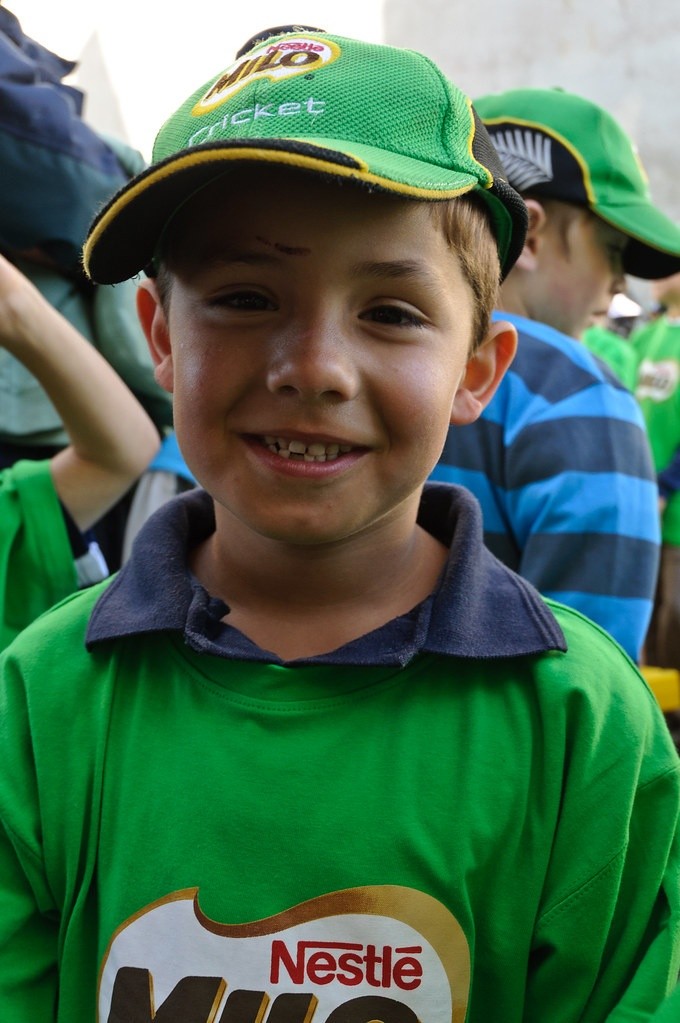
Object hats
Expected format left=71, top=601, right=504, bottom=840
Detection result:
left=467, top=85, right=680, bottom=281
left=81, top=28, right=527, bottom=286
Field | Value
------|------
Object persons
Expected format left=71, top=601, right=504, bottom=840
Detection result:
left=425, top=84, right=680, bottom=674
left=0, top=27, right=680, bottom=1023
left=0, top=256, right=161, bottom=663
left=0, top=6, right=174, bottom=467
